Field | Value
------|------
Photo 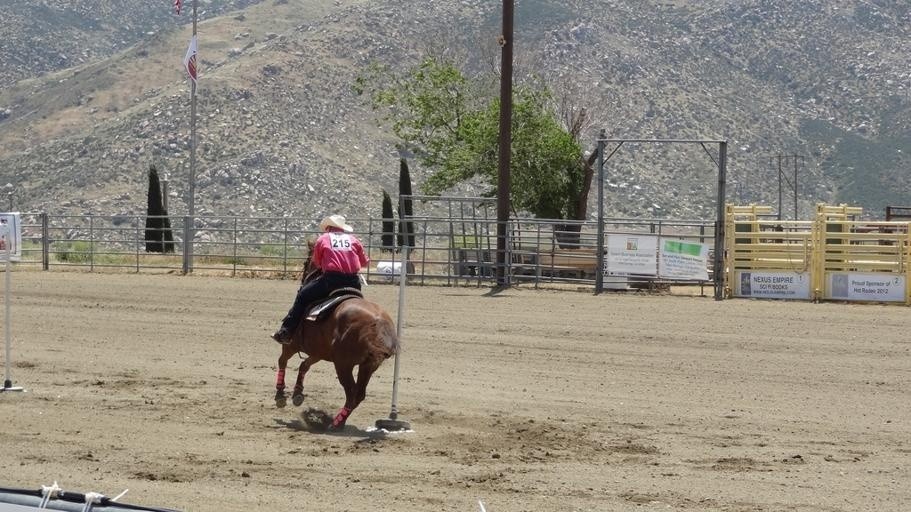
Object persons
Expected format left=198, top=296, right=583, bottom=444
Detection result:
left=270, top=216, right=369, bottom=345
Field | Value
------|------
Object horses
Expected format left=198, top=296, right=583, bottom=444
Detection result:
left=275, top=240, right=398, bottom=432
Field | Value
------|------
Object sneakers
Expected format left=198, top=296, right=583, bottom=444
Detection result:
left=274, top=329, right=291, bottom=344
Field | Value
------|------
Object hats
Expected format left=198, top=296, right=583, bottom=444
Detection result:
left=320, top=215, right=354, bottom=233
